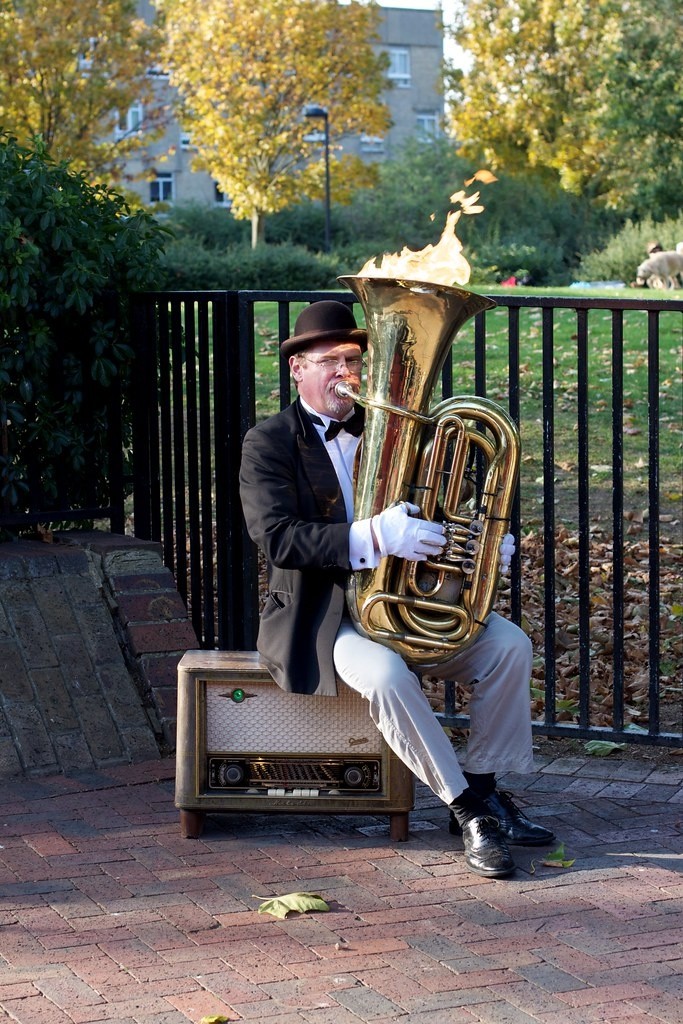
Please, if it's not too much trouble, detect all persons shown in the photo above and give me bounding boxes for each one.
[630,242,683,289]
[240,300,556,876]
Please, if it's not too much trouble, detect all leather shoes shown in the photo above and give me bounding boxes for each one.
[462,811,516,877]
[483,790,557,845]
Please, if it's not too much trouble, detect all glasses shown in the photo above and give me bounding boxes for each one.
[299,355,368,373]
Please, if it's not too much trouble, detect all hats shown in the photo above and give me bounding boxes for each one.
[280,299,369,362]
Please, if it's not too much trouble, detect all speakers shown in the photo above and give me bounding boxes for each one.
[174,648,414,812]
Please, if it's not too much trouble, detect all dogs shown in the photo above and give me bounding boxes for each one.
[636,242,683,290]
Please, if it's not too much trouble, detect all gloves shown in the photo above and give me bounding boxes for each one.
[499,534,516,574]
[372,501,448,561]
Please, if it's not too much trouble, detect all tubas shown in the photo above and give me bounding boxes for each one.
[329,274,523,668]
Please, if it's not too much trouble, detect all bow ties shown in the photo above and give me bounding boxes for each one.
[304,409,364,442]
